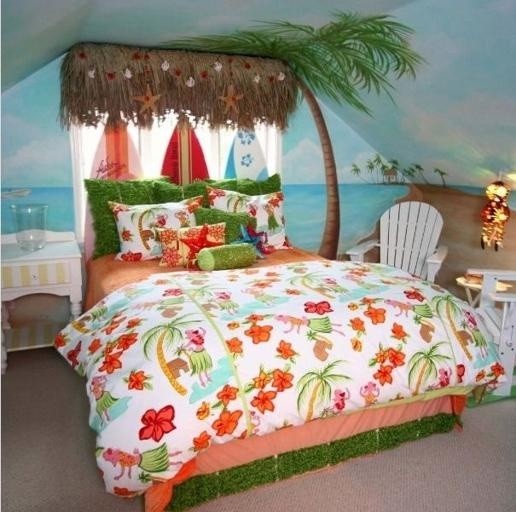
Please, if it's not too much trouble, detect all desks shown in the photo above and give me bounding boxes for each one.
[456,275,508,309]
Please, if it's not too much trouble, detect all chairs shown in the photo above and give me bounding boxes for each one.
[344,200,450,284]
[472,271,516,397]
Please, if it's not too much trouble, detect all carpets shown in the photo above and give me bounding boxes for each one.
[465,367,516,409]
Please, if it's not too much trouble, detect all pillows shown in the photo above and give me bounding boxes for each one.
[82,173,288,273]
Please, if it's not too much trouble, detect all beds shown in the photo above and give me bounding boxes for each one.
[86,247,478,510]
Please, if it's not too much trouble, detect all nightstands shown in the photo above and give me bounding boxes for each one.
[0,228,82,376]
